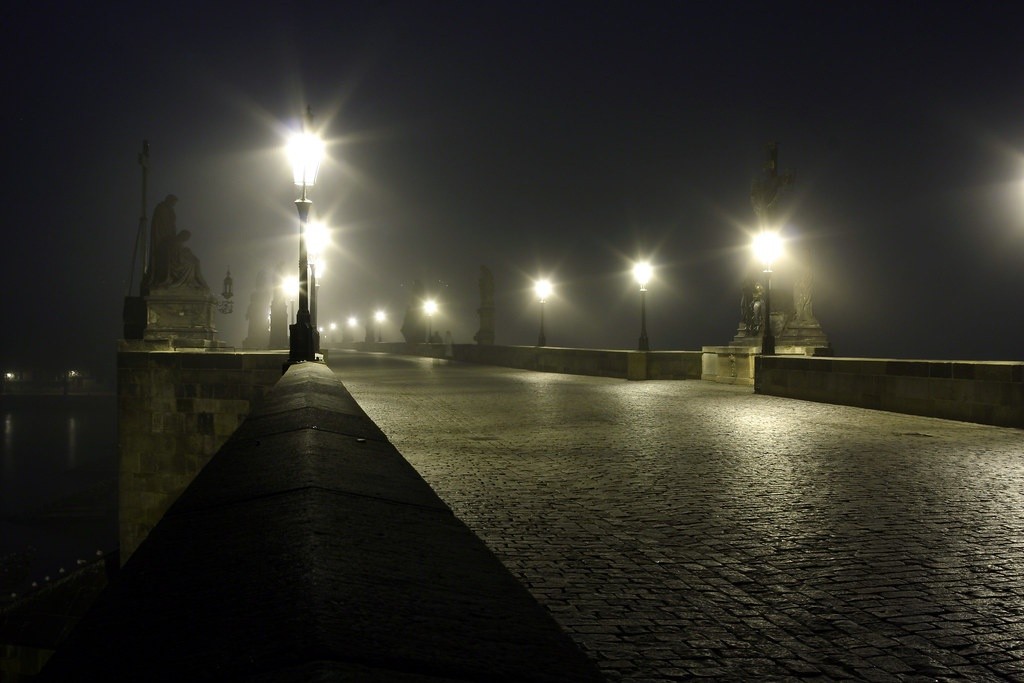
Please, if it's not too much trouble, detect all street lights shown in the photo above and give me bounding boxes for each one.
[287,222,325,354]
[533,278,553,347]
[282,130,326,361]
[422,300,437,344]
[751,229,785,356]
[374,310,385,343]
[631,260,654,351]
